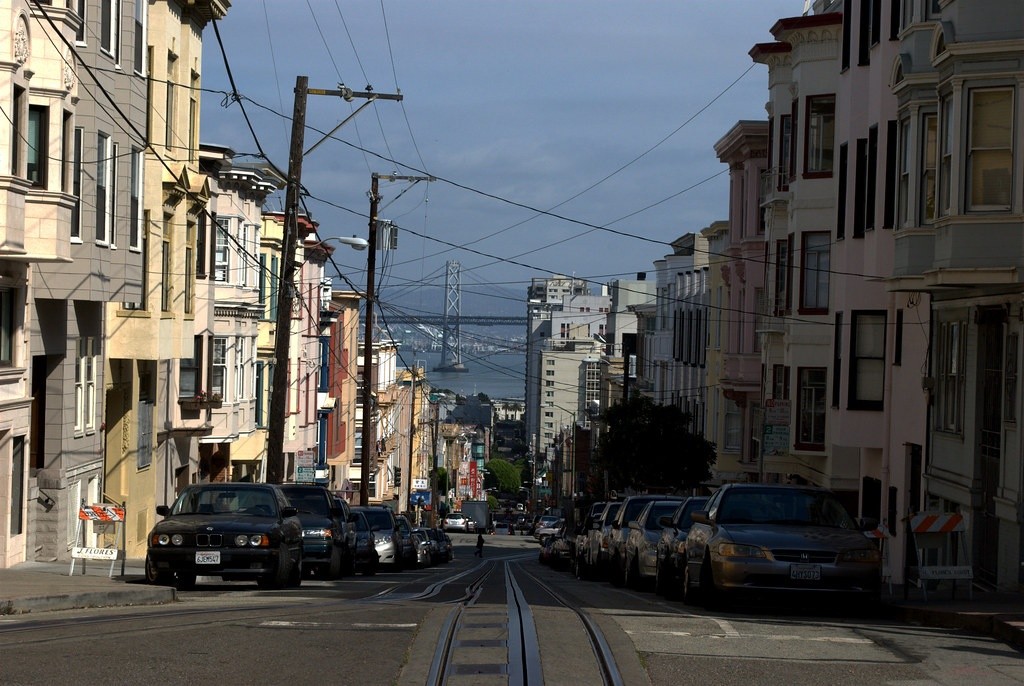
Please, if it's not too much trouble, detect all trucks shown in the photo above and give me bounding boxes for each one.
[460,500,494,534]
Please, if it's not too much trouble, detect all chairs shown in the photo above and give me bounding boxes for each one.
[197,503,214,512]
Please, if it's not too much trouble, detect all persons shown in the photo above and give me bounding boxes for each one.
[474,534,485,558]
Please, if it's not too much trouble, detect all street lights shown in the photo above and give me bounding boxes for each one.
[264,237,368,490]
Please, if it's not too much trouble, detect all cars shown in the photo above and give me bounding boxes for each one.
[516,502,523,511]
[145,482,304,592]
[536,477,881,623]
[517,516,524,522]
[271,480,455,582]
[442,512,480,533]
[525,520,532,529]
